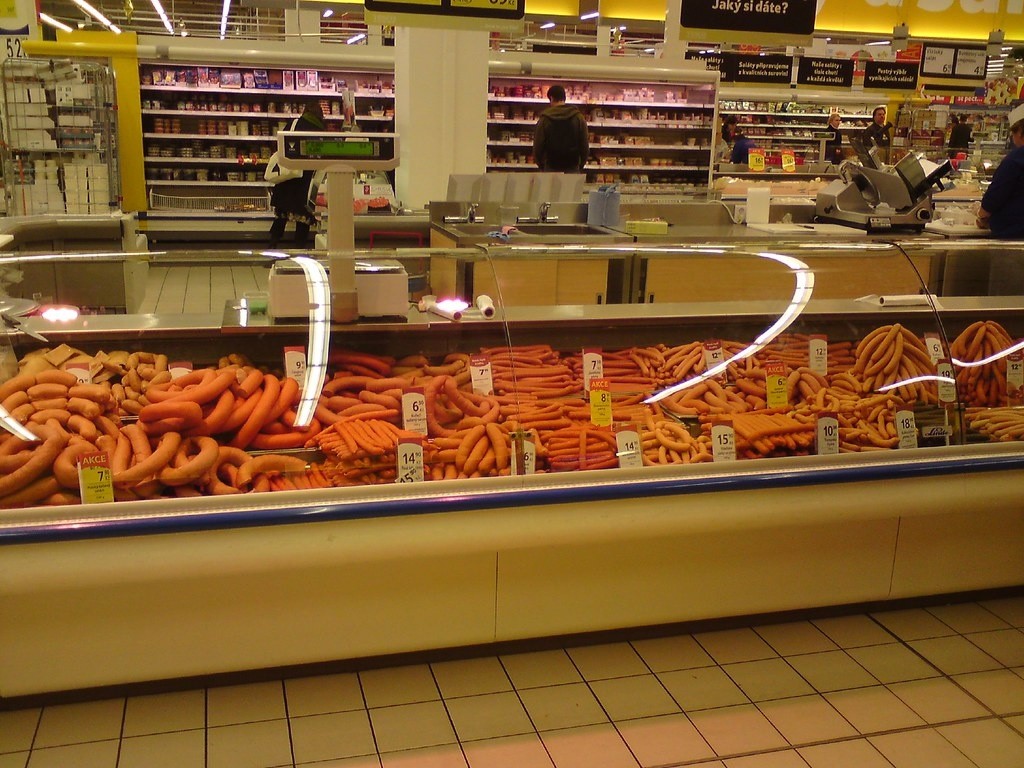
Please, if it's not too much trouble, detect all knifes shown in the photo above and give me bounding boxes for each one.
[797,225,814,229]
[1,313,49,342]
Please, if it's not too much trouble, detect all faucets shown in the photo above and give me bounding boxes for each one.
[517,201,558,223]
[443,201,485,224]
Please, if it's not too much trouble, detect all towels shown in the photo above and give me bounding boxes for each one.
[483,225,518,241]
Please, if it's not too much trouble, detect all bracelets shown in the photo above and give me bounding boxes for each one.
[977,208,988,224]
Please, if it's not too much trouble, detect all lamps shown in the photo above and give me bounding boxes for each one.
[987,30,1005,57]
[579,0,600,21]
[178,17,184,25]
[793,47,804,58]
[892,24,909,52]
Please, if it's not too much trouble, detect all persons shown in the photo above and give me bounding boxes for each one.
[944,114,975,159]
[532,84,589,173]
[721,115,757,164]
[382,114,395,195]
[862,107,890,169]
[817,114,842,165]
[976,101,1024,240]
[264,102,327,249]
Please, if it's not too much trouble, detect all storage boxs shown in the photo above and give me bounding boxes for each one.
[895,107,947,147]
[0,60,115,216]
[626,220,669,235]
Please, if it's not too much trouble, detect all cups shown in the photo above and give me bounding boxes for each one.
[746,187,770,224]
[244,292,269,313]
[500,205,519,227]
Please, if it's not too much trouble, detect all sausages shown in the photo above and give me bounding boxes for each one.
[0,321,1024,514]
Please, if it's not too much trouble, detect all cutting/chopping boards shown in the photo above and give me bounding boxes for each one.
[925,224,991,233]
[747,224,867,234]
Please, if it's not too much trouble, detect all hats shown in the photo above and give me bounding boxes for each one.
[1008,103,1024,128]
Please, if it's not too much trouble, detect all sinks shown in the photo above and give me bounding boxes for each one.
[452,225,520,235]
[512,225,612,235]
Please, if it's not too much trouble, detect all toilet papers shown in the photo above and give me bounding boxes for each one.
[746,187,770,223]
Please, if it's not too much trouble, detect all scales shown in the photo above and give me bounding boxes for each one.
[267,129,410,323]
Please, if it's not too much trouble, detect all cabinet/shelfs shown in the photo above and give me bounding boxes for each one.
[141,85,395,213]
[720,110,886,154]
[947,120,1010,147]
[487,98,715,195]
[463,245,1024,308]
[0,55,114,217]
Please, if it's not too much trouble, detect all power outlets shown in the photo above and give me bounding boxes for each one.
[734,205,746,225]
